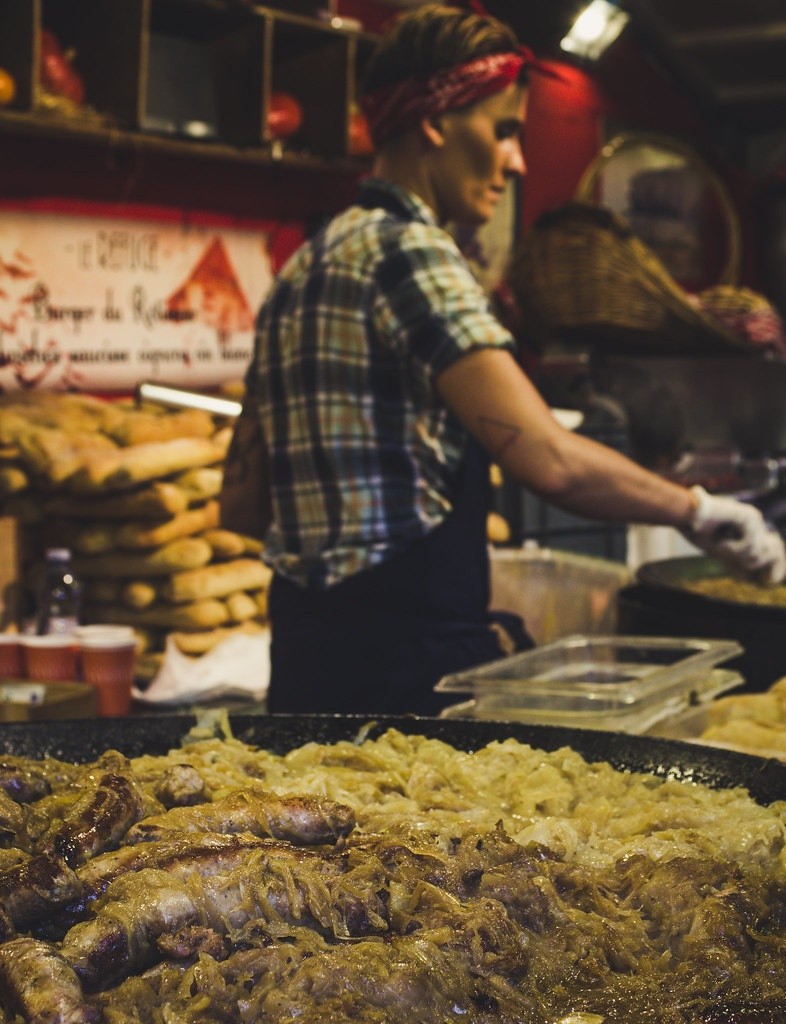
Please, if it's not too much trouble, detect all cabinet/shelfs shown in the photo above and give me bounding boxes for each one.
[0,1,376,180]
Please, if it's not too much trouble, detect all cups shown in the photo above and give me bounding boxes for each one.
[1,635,28,681]
[82,634,137,716]
[26,634,81,717]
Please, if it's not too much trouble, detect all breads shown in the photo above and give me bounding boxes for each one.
[0,377,511,679]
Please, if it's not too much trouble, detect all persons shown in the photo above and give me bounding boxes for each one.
[220,0,786,716]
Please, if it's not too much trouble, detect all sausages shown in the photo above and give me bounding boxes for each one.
[0,751,452,1024]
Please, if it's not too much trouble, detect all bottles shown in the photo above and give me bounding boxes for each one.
[35,549,81,634]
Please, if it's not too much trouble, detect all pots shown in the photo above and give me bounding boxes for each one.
[637,555,786,628]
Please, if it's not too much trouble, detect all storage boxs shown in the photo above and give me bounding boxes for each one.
[430,632,745,733]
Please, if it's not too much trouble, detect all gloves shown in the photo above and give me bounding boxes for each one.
[680,483,786,586]
